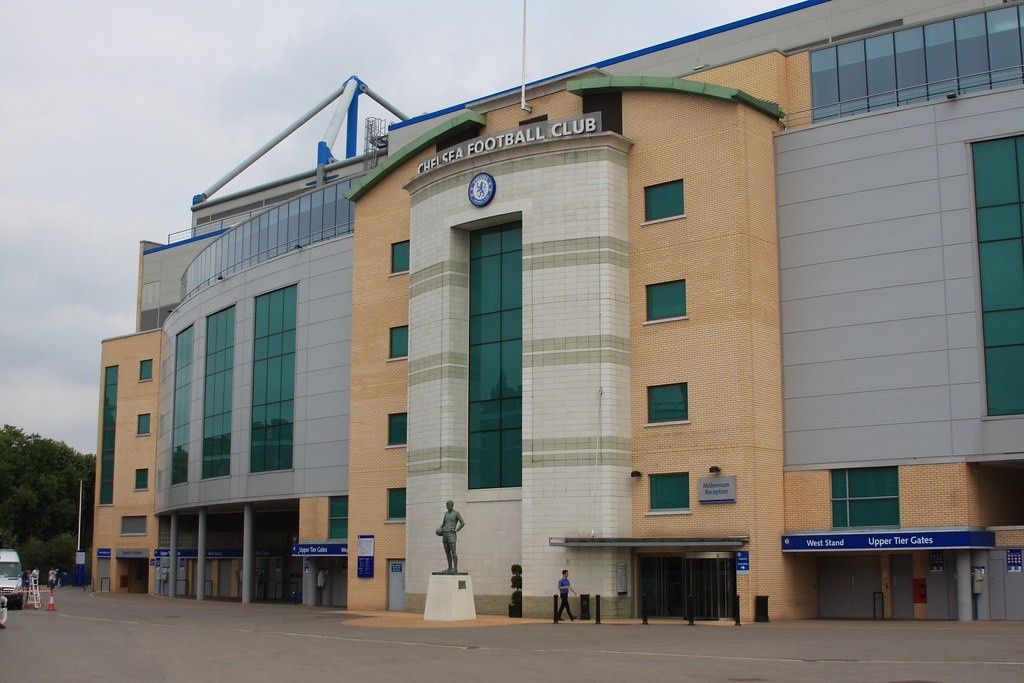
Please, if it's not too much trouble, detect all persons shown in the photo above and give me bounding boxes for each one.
[436,499,465,572]
[26,568,39,588]
[557,570,577,621]
[48,567,57,589]
[60,570,67,587]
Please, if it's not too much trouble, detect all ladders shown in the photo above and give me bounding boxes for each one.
[24,570,42,611]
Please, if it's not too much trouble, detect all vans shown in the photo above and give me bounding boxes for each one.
[0,548,27,610]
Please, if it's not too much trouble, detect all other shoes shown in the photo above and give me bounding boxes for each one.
[558,618,563,621]
[571,616,577,621]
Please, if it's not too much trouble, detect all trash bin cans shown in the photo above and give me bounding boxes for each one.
[580,594,591,620]
[755,595,770,622]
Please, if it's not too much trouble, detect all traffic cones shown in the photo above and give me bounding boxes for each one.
[46,589,58,612]
